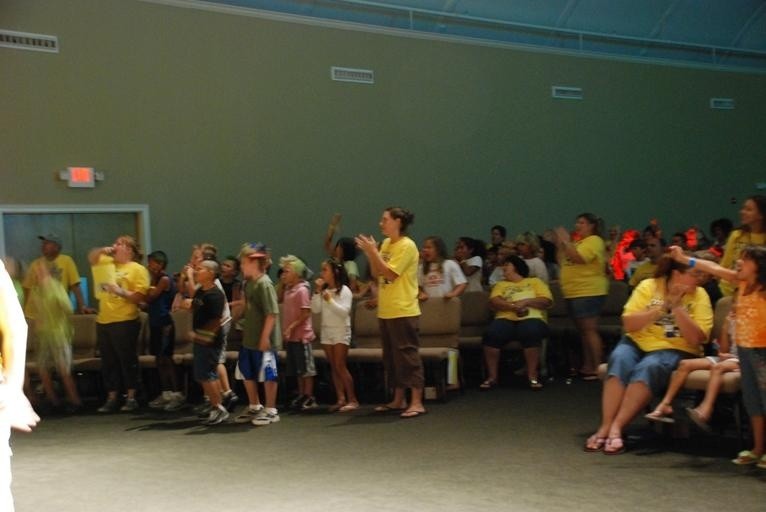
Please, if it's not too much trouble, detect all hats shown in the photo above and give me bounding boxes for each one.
[38,233,63,245]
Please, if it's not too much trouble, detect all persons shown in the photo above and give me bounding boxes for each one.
[235,240,285,426]
[354,206,428,418]
[274,255,319,411]
[87,236,151,413]
[479,255,555,390]
[583,249,714,456]
[556,212,609,380]
[514,229,549,378]
[0,231,97,512]
[138,244,236,427]
[415,224,643,302]
[310,256,360,412]
[644,192,766,471]
[236,212,380,314]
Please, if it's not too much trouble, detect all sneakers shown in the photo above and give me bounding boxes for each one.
[97,390,359,426]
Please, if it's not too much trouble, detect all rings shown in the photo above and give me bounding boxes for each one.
[512,303,514,306]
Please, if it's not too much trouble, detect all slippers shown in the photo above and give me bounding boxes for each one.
[374,405,406,412]
[479,378,496,390]
[528,376,542,389]
[399,408,427,418]
[583,401,765,469]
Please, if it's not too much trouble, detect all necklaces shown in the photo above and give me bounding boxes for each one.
[115,263,124,273]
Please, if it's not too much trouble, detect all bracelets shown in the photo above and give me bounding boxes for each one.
[120,288,125,298]
